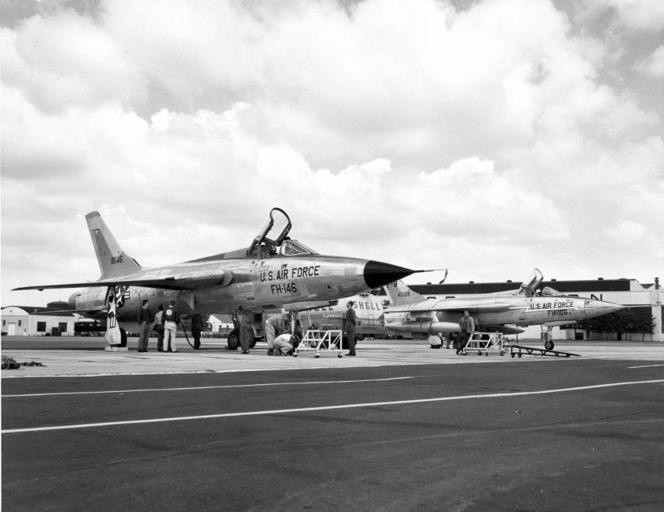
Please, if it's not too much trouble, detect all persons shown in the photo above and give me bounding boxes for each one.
[289,319,302,341]
[237,304,254,354]
[343,300,356,357]
[455,310,475,355]
[137,300,151,352]
[310,323,324,348]
[446,332,457,349]
[150,303,165,352]
[190,312,203,350]
[159,299,179,352]
[272,333,300,355]
[264,312,291,356]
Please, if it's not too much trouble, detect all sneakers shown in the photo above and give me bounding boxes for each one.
[267,348,294,356]
[346,353,353,356]
[158,347,178,353]
[138,349,147,352]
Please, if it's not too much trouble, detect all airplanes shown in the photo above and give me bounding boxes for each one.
[10,207,426,351]
[282,268,634,356]
